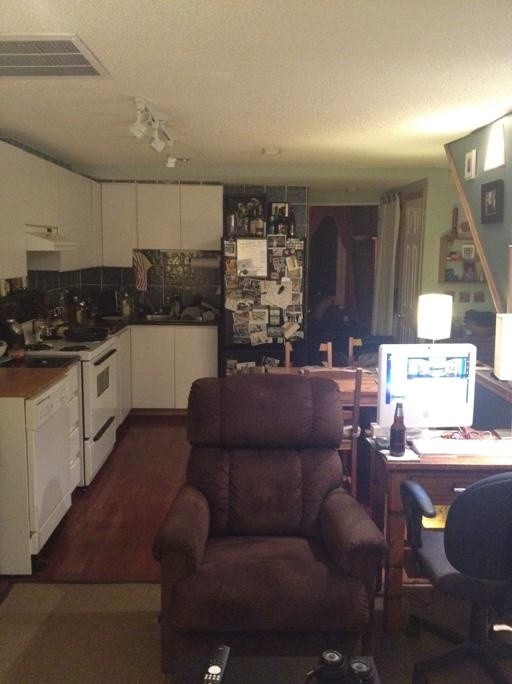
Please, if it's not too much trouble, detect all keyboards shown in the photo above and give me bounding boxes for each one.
[410,438,512,457]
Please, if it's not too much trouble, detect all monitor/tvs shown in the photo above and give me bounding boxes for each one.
[378,343,477,440]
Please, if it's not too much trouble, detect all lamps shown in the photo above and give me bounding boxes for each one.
[127,98,177,171]
[494,313,512,382]
[415,292,453,342]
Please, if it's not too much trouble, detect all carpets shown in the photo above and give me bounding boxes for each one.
[1,578,510,684]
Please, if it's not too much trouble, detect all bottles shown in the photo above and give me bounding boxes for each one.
[389,403,407,457]
[173,295,180,320]
[73,295,89,325]
[120,293,132,320]
[249,204,296,236]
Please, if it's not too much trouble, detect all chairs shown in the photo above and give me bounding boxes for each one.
[399,472,512,668]
[284,342,332,366]
[303,368,363,501]
[347,336,395,368]
[152,374,390,680]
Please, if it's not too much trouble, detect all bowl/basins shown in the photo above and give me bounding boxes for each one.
[64,326,108,341]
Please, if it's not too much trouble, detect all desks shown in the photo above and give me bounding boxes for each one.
[268,365,380,407]
[366,424,512,629]
[171,651,382,684]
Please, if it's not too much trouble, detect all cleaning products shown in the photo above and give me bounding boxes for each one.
[123,293,132,316]
[173,294,182,318]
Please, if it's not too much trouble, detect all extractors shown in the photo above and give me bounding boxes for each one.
[25,224,77,253]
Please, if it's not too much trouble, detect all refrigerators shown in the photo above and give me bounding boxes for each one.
[216,235,308,379]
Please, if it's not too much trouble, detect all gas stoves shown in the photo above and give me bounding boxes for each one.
[17,331,117,360]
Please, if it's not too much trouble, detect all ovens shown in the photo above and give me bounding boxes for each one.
[81,345,118,490]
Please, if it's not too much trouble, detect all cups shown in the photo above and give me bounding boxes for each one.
[1,341,6,358]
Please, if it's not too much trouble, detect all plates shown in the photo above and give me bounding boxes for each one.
[0,354,12,364]
